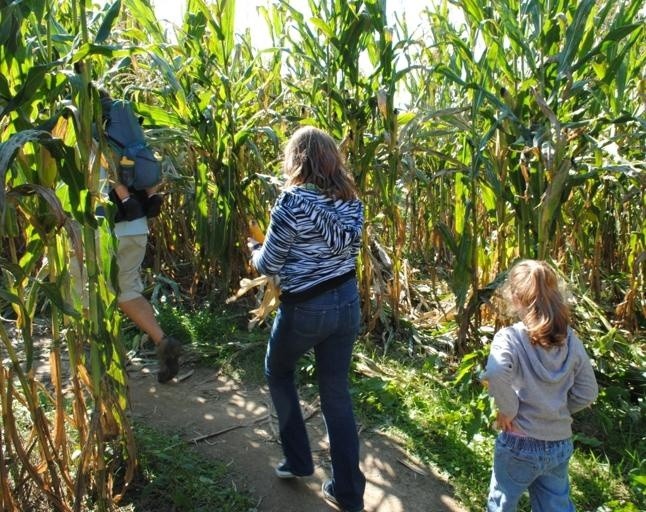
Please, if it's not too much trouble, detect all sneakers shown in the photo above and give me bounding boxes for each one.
[321,479,364,511]
[122,198,140,221]
[275,462,313,478]
[158,337,181,382]
[147,194,161,218]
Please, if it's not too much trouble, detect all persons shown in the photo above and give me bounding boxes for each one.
[57,89,181,384]
[248,126,365,512]
[486,260,598,512]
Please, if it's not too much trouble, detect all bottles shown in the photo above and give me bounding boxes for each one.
[118,153,137,187]
